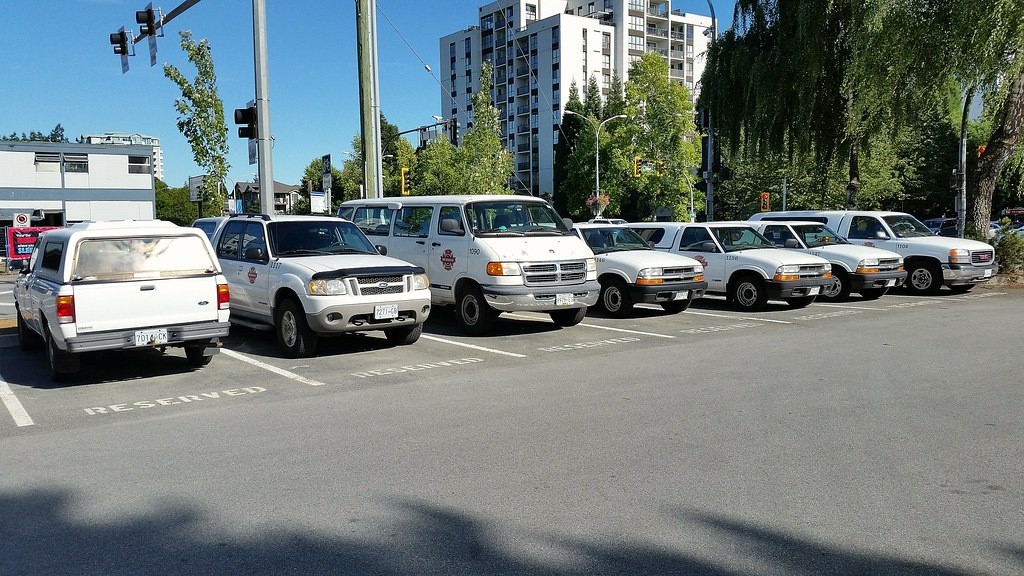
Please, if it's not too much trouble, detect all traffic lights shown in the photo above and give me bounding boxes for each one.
[401,167,411,195]
[760,192,769,211]
[451,118,460,142]
[110,31,128,55]
[419,126,427,150]
[978,145,986,157]
[136,9,155,36]
[702,135,720,173]
[235,107,256,139]
[656,160,664,177]
[634,156,642,177]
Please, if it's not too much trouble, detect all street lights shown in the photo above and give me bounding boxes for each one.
[564,109,627,219]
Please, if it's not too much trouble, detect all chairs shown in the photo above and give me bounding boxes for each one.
[493,214,510,229]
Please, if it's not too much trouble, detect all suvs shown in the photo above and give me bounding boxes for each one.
[923,217,958,238]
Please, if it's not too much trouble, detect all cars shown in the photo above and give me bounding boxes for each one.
[588,219,628,226]
[987,219,1024,243]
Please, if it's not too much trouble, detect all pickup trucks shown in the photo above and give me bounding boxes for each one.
[12,218,230,382]
[510,217,708,318]
[748,211,999,295]
[191,213,431,358]
[628,222,834,311]
[715,222,908,303]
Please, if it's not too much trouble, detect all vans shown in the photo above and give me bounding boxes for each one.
[337,194,602,335]
